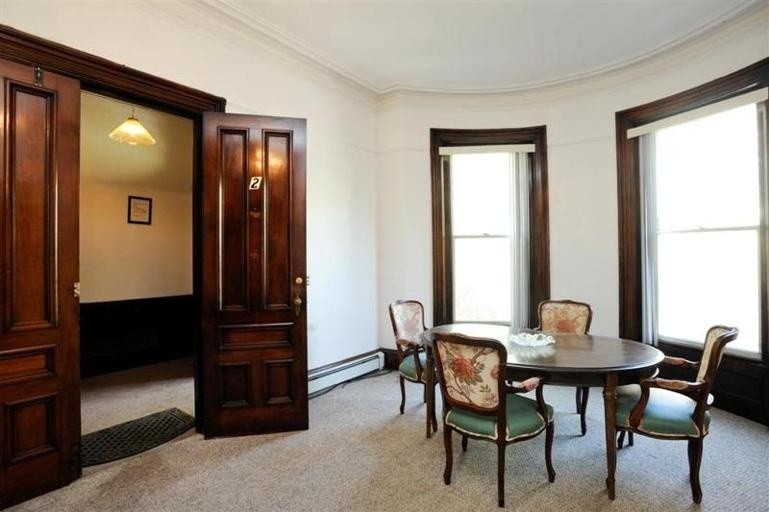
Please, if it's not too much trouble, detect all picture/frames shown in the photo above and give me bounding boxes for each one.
[125,195,152,226]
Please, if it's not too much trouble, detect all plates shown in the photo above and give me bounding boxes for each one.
[506,345,556,359]
[504,328,553,345]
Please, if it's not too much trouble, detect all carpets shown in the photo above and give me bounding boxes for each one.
[77,407,195,463]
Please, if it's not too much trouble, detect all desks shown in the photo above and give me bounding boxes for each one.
[425,322,667,486]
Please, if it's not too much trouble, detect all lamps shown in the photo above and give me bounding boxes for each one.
[106,106,157,148]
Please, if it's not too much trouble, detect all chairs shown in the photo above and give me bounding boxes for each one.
[604,321,742,505]
[529,298,595,436]
[427,330,559,508]
[388,296,443,432]
